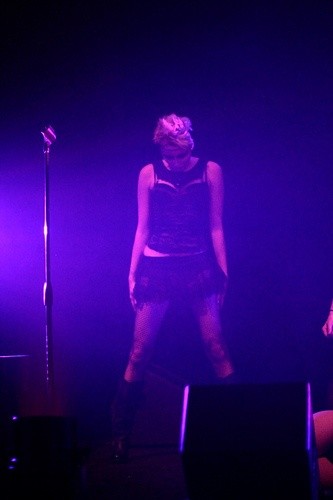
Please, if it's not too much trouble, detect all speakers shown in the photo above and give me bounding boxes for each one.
[177,380,321,500]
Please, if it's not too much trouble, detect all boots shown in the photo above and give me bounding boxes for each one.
[111,381,143,464]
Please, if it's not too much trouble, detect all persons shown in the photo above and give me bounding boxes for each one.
[108,113,233,463]
